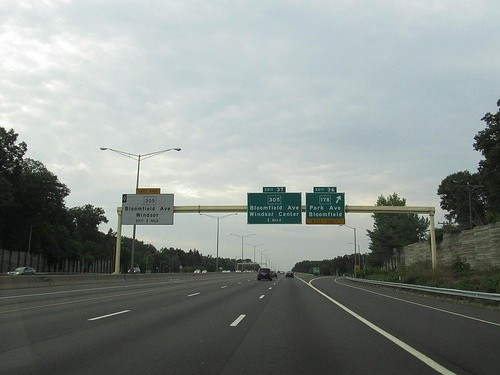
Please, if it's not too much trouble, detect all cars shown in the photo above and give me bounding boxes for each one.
[7,267,36,276]
[286,271,294,278]
[128,268,141,273]
[194,270,207,274]
[271,271,277,278]
[280,271,284,274]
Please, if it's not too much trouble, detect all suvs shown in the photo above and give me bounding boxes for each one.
[257,267,273,281]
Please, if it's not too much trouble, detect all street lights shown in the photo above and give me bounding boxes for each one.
[246,243,264,271]
[230,233,256,272]
[146,254,154,272]
[200,213,238,271]
[160,261,167,272]
[26,222,47,266]
[99,147,181,273]
[340,225,357,277]
[113,242,124,272]
[349,243,361,271]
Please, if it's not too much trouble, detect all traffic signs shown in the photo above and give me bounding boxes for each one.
[305,192,346,225]
